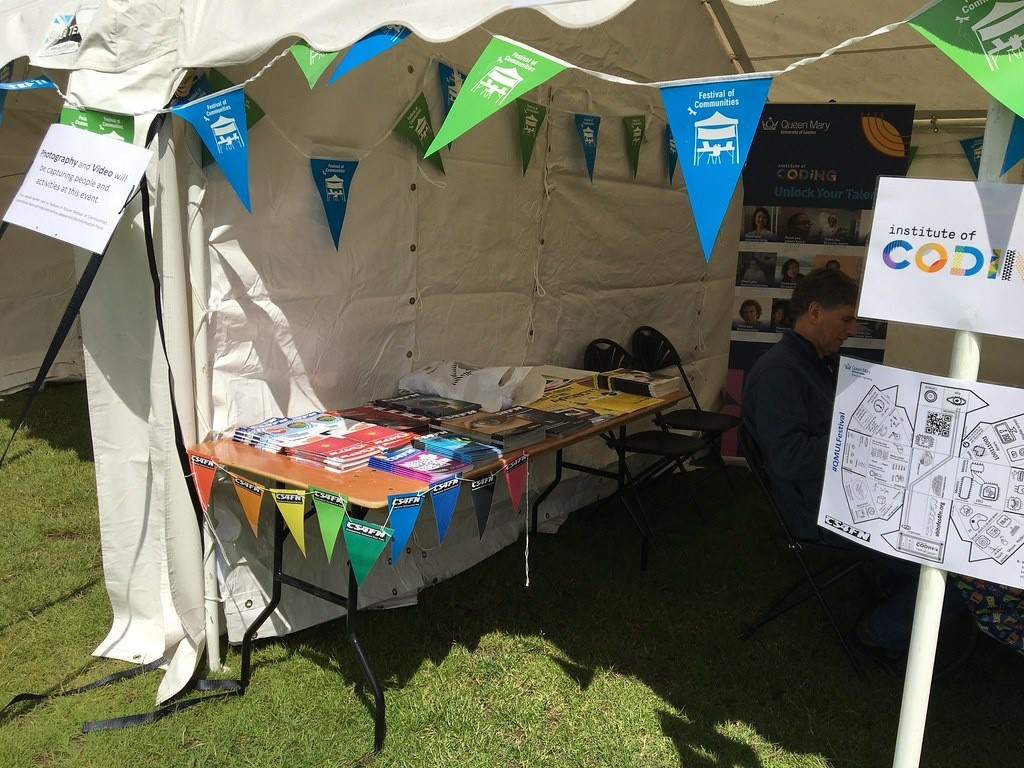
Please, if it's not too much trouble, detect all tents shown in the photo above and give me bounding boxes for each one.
[180,0,1024,768]
[0,0,205,705]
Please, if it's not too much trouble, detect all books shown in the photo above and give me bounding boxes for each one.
[231,364,680,484]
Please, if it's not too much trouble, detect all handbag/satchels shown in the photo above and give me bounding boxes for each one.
[399,360,548,411]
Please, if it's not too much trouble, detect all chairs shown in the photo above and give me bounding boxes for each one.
[734,426,869,681]
[585,337,707,549]
[631,325,741,516]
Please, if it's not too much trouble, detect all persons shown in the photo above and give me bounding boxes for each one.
[771,307,784,329]
[741,207,777,242]
[826,260,841,271]
[778,258,806,289]
[819,214,848,244]
[742,257,766,283]
[738,268,976,686]
[739,299,763,331]
[782,213,814,244]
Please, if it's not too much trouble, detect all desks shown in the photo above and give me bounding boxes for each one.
[932,572,1024,683]
[189,381,691,761]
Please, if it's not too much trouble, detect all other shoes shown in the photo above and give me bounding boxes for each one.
[842,624,904,680]
